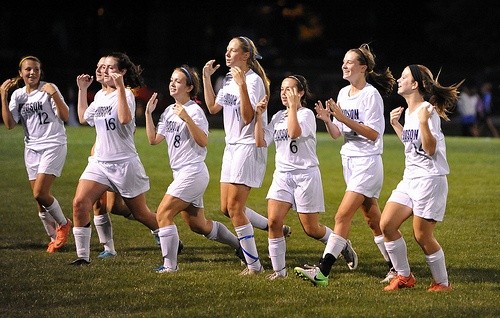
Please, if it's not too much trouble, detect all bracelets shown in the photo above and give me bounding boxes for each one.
[50,91,57,96]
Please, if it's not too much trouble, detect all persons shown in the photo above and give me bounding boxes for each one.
[379,64,449,292]
[202,36,291,275]
[144,66,248,272]
[0,56,74,253]
[87,53,161,258]
[292,42,397,287]
[66,52,184,267]
[254,74,358,279]
[456,84,495,136]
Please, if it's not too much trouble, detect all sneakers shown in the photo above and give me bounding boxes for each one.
[340,238,358,271]
[97,251,118,259]
[427,281,451,293]
[154,265,179,273]
[237,266,265,276]
[265,272,289,281]
[46,241,57,253]
[53,218,74,249]
[379,268,398,284]
[384,273,416,290]
[293,265,329,287]
[68,258,91,266]
[280,225,291,239]
